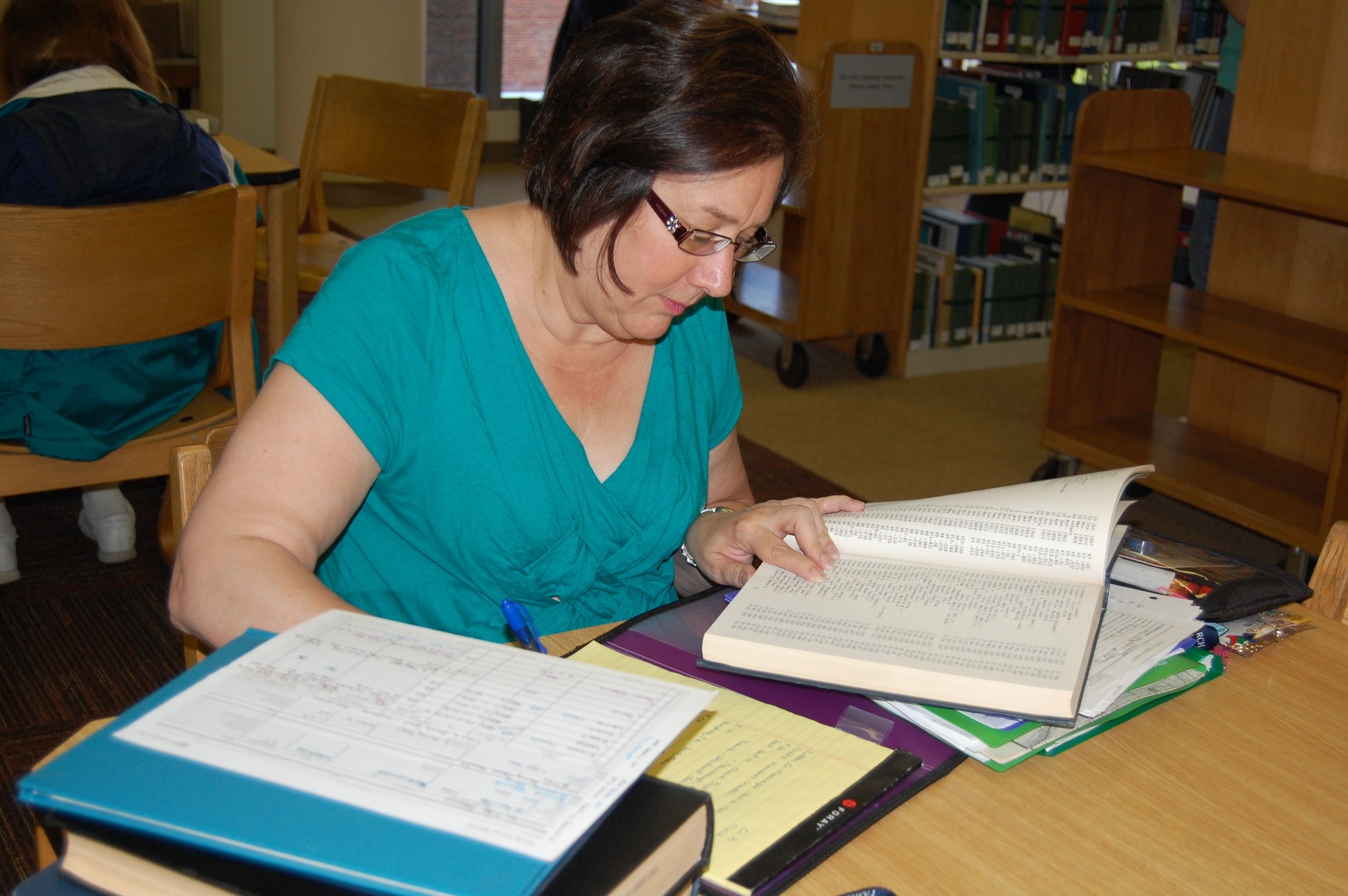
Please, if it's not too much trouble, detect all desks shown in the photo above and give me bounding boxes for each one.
[214,132,301,363]
[28,600,1348,896]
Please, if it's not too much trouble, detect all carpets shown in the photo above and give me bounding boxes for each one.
[0,428,862,896]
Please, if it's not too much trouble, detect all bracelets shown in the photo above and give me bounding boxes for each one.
[681,507,738,567]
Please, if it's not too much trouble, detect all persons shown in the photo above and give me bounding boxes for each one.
[170,5,866,652]
[0,0,264,582]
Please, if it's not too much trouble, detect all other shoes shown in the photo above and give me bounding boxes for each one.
[79,501,137,563]
[0,523,21,584]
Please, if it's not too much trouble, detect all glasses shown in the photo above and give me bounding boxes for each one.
[645,187,776,262]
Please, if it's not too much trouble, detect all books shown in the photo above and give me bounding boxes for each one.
[942,0,1225,56]
[927,63,1232,186]
[909,205,1064,348]
[18,609,919,896]
[694,463,1223,771]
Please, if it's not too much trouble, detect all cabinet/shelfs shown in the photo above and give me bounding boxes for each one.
[902,0,1229,377]
[1029,91,1348,579]
[706,0,930,388]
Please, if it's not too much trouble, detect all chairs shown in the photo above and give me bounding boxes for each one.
[0,183,255,557]
[251,75,490,292]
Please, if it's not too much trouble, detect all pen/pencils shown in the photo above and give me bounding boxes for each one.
[501,599,545,654]
